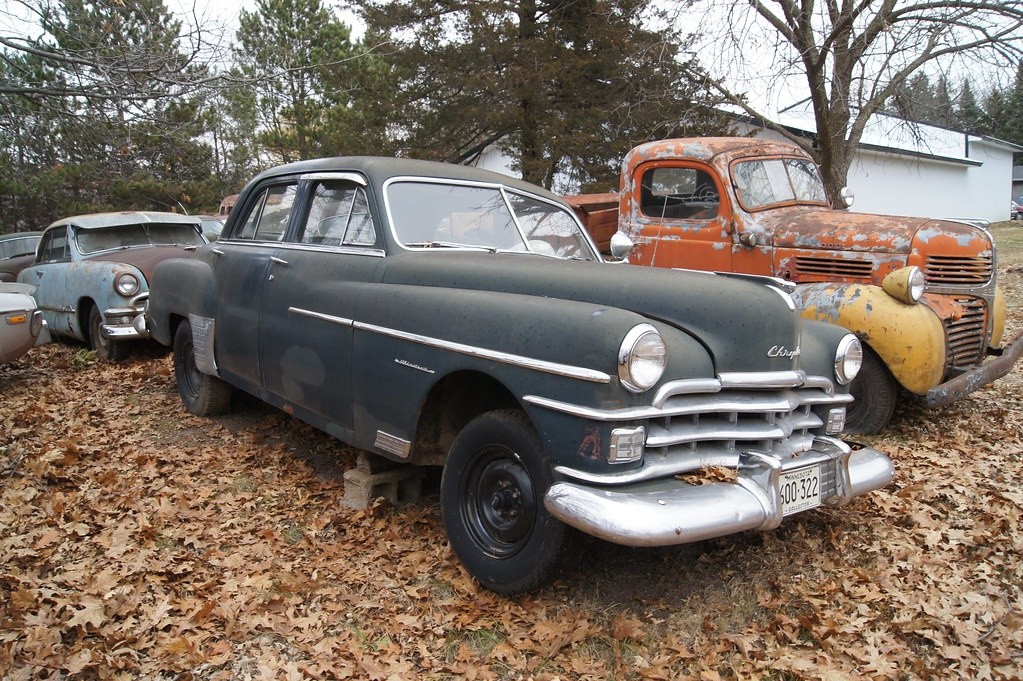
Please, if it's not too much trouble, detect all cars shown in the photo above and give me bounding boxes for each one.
[144,148,897,593]
[14,205,212,367]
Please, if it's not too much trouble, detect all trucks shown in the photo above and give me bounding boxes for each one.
[556,135,1010,440]
